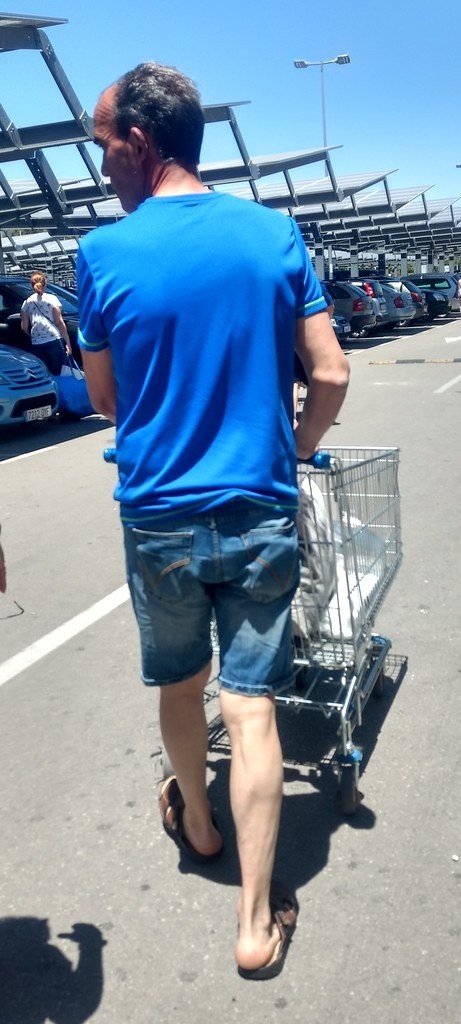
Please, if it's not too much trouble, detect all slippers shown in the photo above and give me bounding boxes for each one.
[159,775,224,863]
[238,888,296,980]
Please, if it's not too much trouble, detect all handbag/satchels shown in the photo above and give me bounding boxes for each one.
[53,353,95,416]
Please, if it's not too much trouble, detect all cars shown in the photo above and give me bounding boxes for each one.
[1,347,60,432]
[380,284,417,333]
[422,289,449,323]
[346,279,388,321]
[329,315,351,343]
[0,277,81,358]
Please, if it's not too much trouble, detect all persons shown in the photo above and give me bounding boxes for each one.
[74,61,350,980]
[458,276,461,316]
[21,274,71,423]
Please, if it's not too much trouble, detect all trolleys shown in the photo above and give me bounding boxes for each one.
[102,445,403,817]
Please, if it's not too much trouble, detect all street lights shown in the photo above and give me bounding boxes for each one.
[294,54,353,178]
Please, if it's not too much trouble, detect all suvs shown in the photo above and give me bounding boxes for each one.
[319,280,379,337]
[407,273,461,319]
[370,277,429,327]
